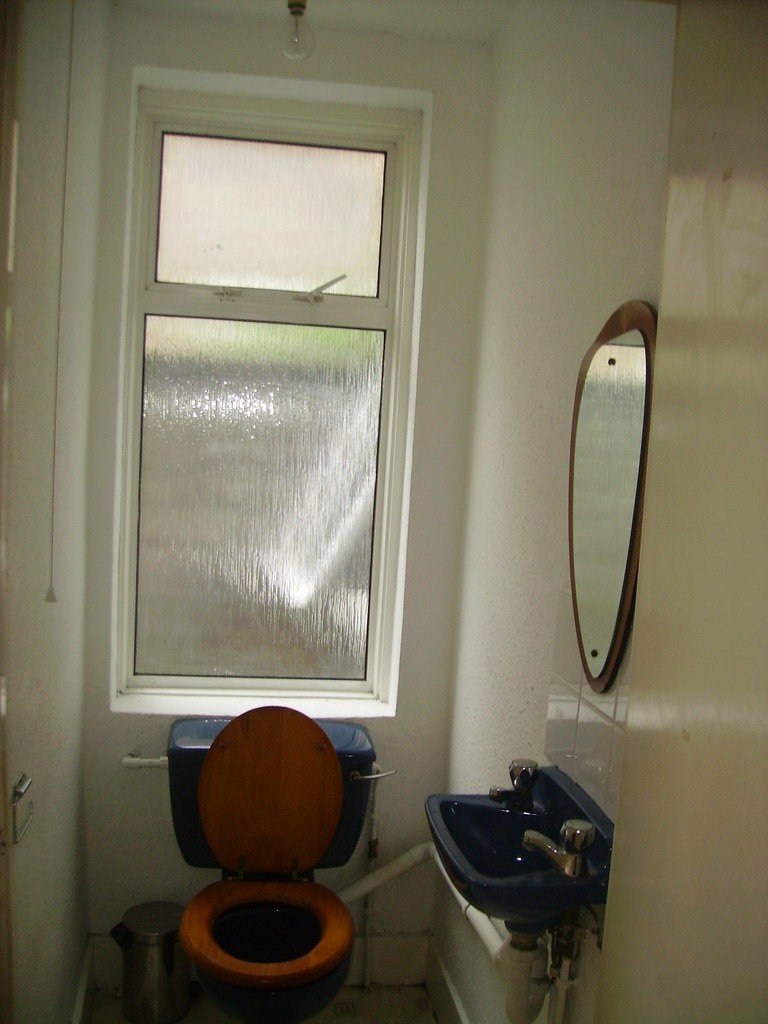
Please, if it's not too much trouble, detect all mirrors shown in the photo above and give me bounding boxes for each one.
[566,297,658,694]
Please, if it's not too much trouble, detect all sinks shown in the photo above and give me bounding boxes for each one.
[424,764,615,923]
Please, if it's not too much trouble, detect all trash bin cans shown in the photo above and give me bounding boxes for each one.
[108,900,192,1024]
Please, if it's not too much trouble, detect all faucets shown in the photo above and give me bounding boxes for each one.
[488,758,540,810]
[522,819,596,879]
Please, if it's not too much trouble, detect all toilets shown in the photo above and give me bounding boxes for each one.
[166,705,377,1024]
